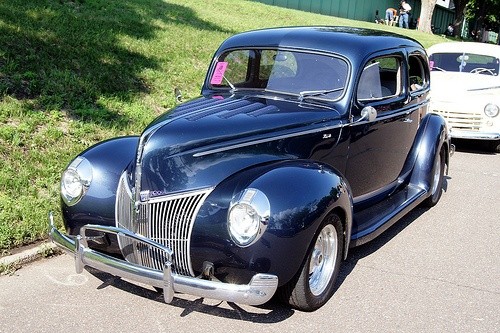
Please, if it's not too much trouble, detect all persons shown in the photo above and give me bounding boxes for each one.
[371,0,456,38]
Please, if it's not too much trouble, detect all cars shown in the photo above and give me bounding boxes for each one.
[47,25,455,312]
[423,41,500,154]
